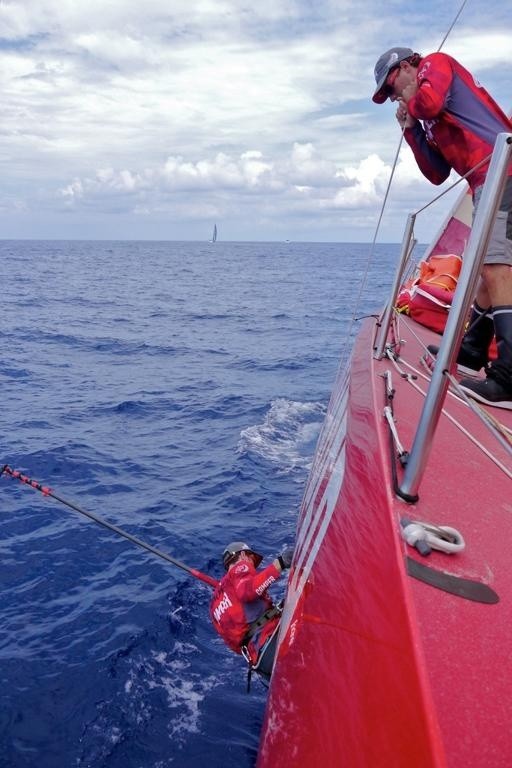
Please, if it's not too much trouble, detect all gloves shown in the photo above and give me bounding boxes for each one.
[278,549,294,571]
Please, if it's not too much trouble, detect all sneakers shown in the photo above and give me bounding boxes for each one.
[427,343,488,376]
[459,367,512,409]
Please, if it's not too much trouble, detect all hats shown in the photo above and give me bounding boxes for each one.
[223,542,263,569]
[371,47,414,104]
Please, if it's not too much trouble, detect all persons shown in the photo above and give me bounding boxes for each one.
[208,541,295,681]
[372,46,512,410]
